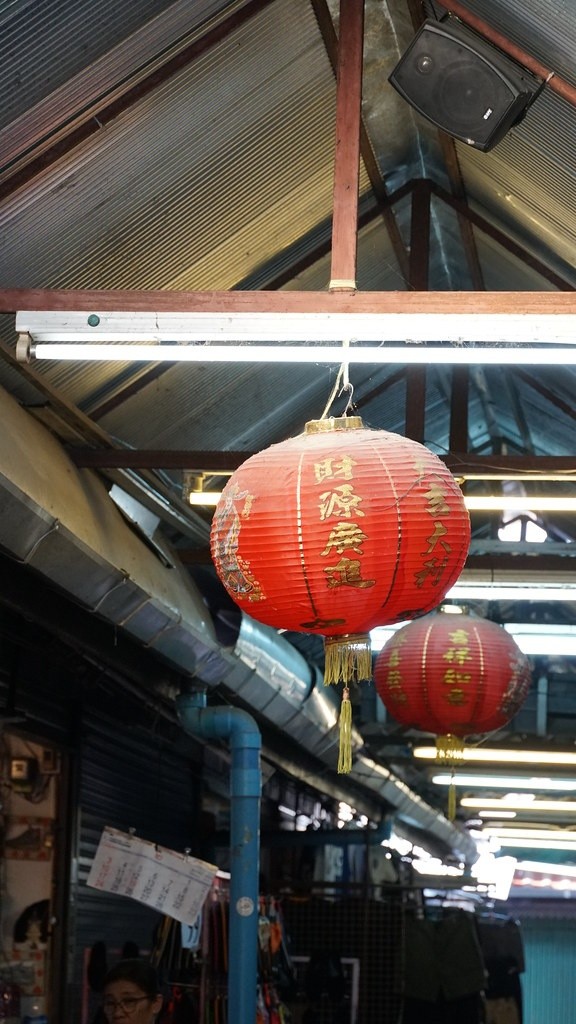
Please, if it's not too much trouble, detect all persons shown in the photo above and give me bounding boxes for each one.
[102,962,168,1024]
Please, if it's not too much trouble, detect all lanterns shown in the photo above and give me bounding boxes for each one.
[209,416,470,774]
[374,604,531,821]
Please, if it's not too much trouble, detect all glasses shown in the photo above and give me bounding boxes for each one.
[101,995,150,1015]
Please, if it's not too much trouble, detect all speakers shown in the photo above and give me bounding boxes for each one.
[390,10,553,153]
